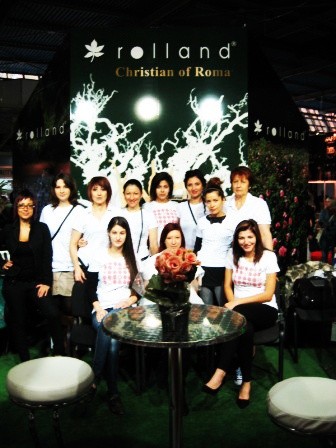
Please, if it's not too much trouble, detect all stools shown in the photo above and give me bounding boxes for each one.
[7,355,96,448]
[266,376,336,448]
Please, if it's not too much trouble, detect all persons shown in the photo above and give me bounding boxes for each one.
[0,191,55,362]
[78,179,159,269]
[206,306,223,325]
[127,306,147,323]
[221,163,275,267]
[139,170,186,255]
[63,215,149,423]
[106,313,122,327]
[189,305,204,323]
[140,222,205,417]
[191,176,237,311]
[174,167,205,256]
[221,310,244,333]
[69,177,131,359]
[316,196,336,266]
[37,175,86,358]
[202,218,283,412]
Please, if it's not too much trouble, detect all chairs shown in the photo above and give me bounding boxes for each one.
[253,279,298,382]
[55,282,98,356]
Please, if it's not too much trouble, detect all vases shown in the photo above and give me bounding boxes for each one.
[159,302,191,341]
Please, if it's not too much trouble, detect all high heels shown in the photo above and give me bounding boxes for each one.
[107,393,124,415]
[79,385,97,404]
[237,390,250,409]
[202,381,222,395]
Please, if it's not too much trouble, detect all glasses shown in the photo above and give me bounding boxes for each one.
[17,205,35,209]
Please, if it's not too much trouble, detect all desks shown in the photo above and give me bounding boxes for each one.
[101,305,246,448]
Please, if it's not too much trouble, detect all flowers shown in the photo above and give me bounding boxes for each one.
[244,142,316,257]
[145,247,201,305]
